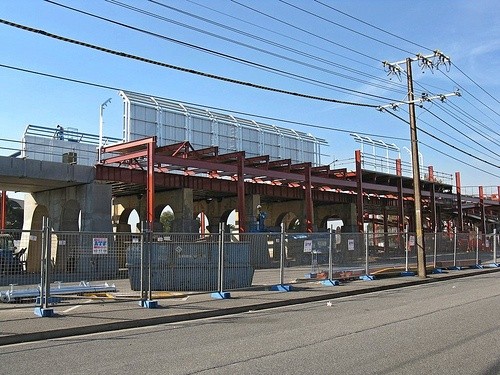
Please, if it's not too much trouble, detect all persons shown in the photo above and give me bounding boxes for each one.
[57,125,64,140]
[8,247,17,264]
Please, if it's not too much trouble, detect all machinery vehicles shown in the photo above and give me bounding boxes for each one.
[0,234,28,274]
